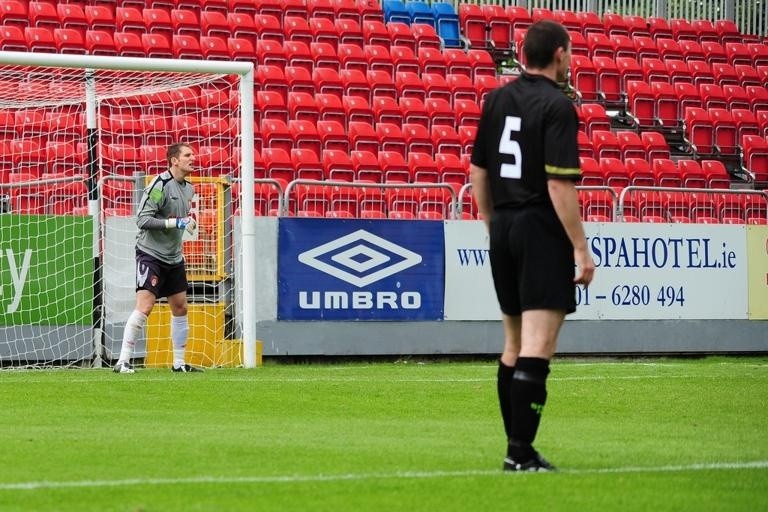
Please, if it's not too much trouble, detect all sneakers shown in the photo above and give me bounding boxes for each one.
[171,363,205,373]
[502,451,556,472]
[113,361,135,375]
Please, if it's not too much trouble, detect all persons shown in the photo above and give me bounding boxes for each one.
[467,21,595,474]
[113,143,202,374]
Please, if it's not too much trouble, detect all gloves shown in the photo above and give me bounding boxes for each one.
[167,216,197,235]
